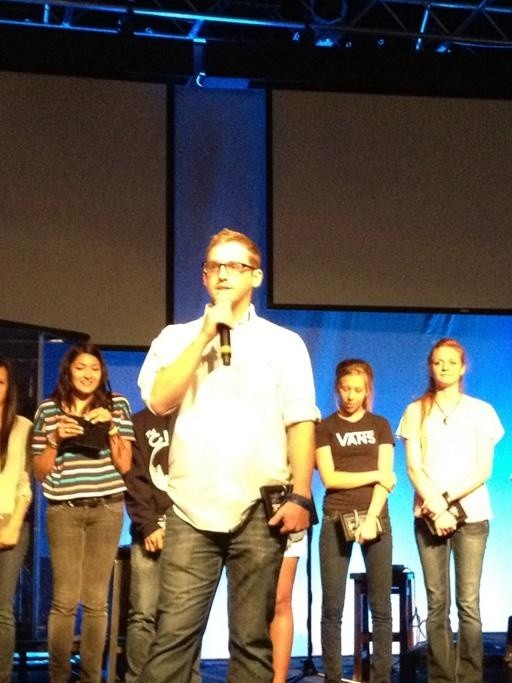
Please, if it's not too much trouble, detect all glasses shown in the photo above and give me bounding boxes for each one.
[201,260,255,277]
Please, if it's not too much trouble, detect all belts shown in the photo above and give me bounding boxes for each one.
[44,494,125,509]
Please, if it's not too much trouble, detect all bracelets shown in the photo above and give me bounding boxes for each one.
[287,493,313,511]
[108,424,118,436]
[442,492,452,506]
[46,432,60,449]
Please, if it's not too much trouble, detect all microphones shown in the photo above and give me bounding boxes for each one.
[215,292,233,365]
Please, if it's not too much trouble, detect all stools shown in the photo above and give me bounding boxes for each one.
[353,570,414,683]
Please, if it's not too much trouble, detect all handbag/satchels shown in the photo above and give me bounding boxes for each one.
[58,412,114,452]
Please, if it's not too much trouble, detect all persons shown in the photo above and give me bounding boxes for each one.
[126,406,202,683]
[32,344,136,683]
[269,529,306,683]
[316,358,397,683]
[137,228,322,683]
[0,356,35,683]
[396,338,505,683]
